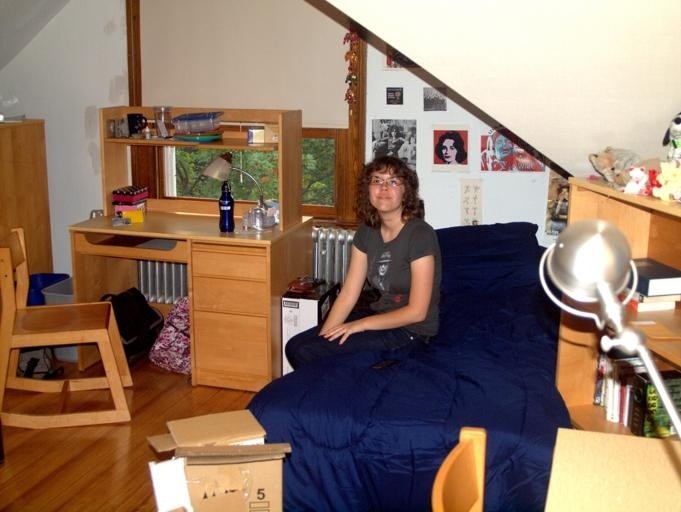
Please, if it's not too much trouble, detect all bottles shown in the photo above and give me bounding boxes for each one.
[155,106,173,138]
[248,207,253,229]
[253,208,263,231]
[145,126,151,140]
[218,180,236,234]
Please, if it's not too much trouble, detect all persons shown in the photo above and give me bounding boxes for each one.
[288,155,446,369]
[434,132,467,165]
[383,125,403,163]
[550,185,569,233]
[481,131,515,170]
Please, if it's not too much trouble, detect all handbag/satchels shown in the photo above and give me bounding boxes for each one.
[100,286,163,360]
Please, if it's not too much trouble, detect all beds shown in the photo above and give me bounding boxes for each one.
[245,221,572,512]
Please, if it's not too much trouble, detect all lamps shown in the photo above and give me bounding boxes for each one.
[538,218,680,442]
[202,150,276,230]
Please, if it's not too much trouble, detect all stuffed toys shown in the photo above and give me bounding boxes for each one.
[579,112,681,207]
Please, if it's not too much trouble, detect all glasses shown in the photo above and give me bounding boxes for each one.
[370,176,402,188]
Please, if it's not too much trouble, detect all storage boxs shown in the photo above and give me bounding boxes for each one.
[41,277,74,306]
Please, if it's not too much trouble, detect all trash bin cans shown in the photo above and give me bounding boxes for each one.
[46,276,78,362]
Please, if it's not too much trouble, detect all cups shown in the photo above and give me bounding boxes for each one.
[128,112,147,136]
[153,106,171,138]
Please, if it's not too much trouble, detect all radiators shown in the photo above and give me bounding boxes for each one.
[311,226,360,283]
[139,259,188,305]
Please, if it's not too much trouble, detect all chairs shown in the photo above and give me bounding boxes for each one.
[431,427,488,511]
[0,225,134,429]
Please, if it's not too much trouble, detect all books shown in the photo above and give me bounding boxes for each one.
[618,257,681,313]
[588,345,681,440]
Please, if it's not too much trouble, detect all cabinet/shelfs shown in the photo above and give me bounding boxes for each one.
[556,176,681,432]
[0,119,56,277]
[68,106,315,393]
